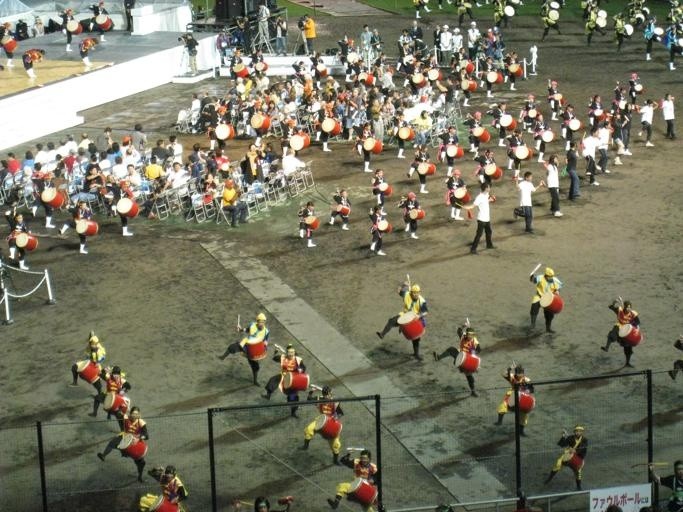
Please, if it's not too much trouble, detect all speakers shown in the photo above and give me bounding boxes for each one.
[227,0,244,16]
[216,0,227,22]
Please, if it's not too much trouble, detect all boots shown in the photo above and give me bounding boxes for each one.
[7,58,14,67]
[376,323,584,490]
[69,372,145,484]
[26,68,36,80]
[191,0,683,256]
[62,23,107,72]
[528,314,679,380]
[216,349,342,510]
[8,206,133,270]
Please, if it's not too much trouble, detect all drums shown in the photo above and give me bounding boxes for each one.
[596,10,608,28]
[321,117,341,137]
[593,108,606,120]
[529,109,543,122]
[653,28,665,42]
[619,99,628,110]
[508,391,536,412]
[290,132,310,151]
[378,182,393,197]
[378,220,393,232]
[215,124,234,140]
[542,131,557,143]
[499,6,514,19]
[634,84,644,94]
[66,20,82,34]
[500,114,517,131]
[540,292,563,314]
[473,127,491,143]
[363,137,383,155]
[618,325,643,345]
[484,163,502,179]
[623,7,650,37]
[77,359,182,512]
[304,216,320,229]
[15,188,139,251]
[454,187,471,204]
[567,119,583,131]
[250,114,271,130]
[397,312,425,341]
[412,72,427,88]
[461,80,478,91]
[563,451,585,470]
[456,351,480,372]
[1,36,18,53]
[255,62,268,72]
[412,59,523,91]
[555,94,565,107]
[416,163,436,175]
[410,209,425,220]
[233,64,248,78]
[516,146,534,160]
[96,14,111,30]
[397,126,415,141]
[336,204,351,218]
[548,2,560,20]
[246,336,380,505]
[358,72,374,86]
[446,144,464,158]
[316,64,327,77]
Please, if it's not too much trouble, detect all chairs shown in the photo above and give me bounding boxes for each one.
[0,135,314,226]
[166,77,468,155]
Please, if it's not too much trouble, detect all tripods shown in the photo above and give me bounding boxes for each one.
[179,46,189,73]
[292,31,309,56]
